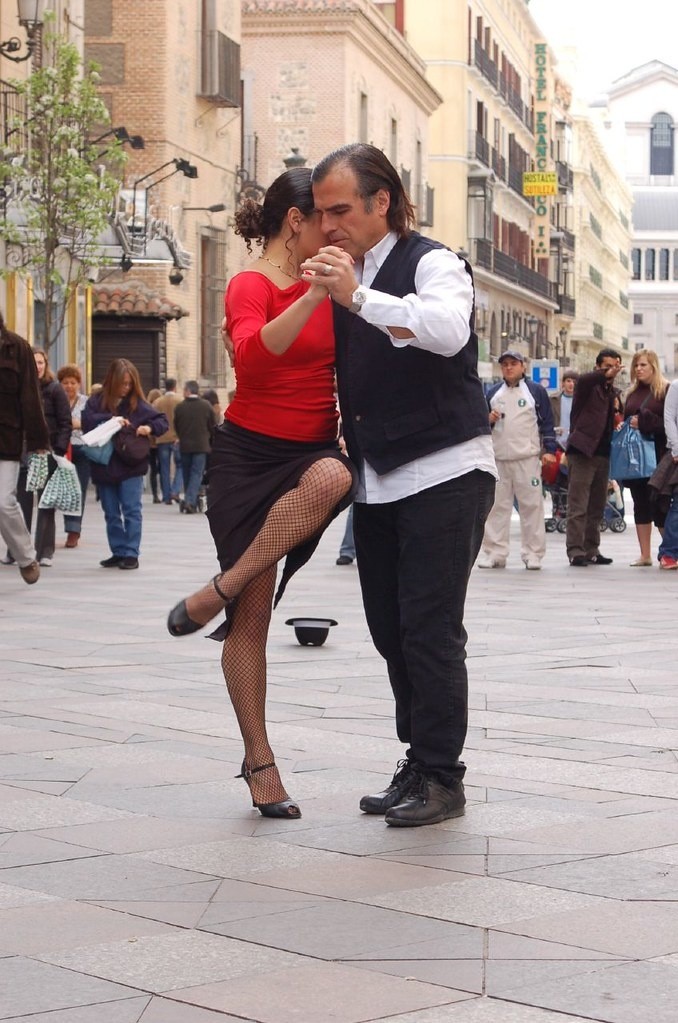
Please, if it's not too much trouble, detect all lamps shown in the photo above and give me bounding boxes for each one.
[143,165,199,238]
[21,236,60,266]
[87,135,145,164]
[183,203,227,212]
[510,315,538,342]
[168,268,184,285]
[542,327,567,351]
[0,0,45,62]
[78,126,130,152]
[96,253,134,282]
[131,158,190,250]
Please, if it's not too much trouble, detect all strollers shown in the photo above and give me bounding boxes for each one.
[541,437,628,533]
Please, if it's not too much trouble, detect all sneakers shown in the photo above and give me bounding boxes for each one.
[569,555,612,565]
[660,554,678,569]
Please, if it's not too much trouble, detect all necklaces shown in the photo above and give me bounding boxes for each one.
[258,256,299,281]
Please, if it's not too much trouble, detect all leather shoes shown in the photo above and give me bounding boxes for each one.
[359,760,467,827]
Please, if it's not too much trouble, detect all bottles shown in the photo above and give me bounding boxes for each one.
[494,401,506,431]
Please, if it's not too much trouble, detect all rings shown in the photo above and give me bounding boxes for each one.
[324,265,333,274]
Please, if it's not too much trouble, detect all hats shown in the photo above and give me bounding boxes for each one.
[498,351,525,364]
[563,370,578,379]
[285,618,337,646]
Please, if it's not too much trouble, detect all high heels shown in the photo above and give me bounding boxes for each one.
[169,491,183,504]
[233,754,302,819]
[167,572,237,637]
[180,499,203,514]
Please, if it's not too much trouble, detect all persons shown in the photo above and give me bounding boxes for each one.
[0,309,52,585]
[0,347,72,566]
[301,141,500,827]
[336,422,356,566]
[90,378,236,515]
[57,363,89,549]
[549,349,678,570]
[81,359,170,570]
[167,167,359,819]
[478,351,556,570]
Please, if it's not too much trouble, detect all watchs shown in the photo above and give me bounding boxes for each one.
[348,284,367,314]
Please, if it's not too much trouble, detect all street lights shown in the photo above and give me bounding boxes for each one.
[560,328,568,371]
[529,315,538,359]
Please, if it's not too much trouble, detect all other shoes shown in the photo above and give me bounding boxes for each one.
[153,497,160,503]
[39,554,54,566]
[337,556,353,565]
[478,557,506,568]
[630,560,652,566]
[101,555,138,569]
[0,553,16,564]
[526,558,541,570]
[66,532,80,547]
[21,561,40,585]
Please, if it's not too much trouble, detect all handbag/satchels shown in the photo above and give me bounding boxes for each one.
[37,450,82,517]
[81,435,114,466]
[25,451,52,492]
[608,416,657,480]
[114,423,150,468]
